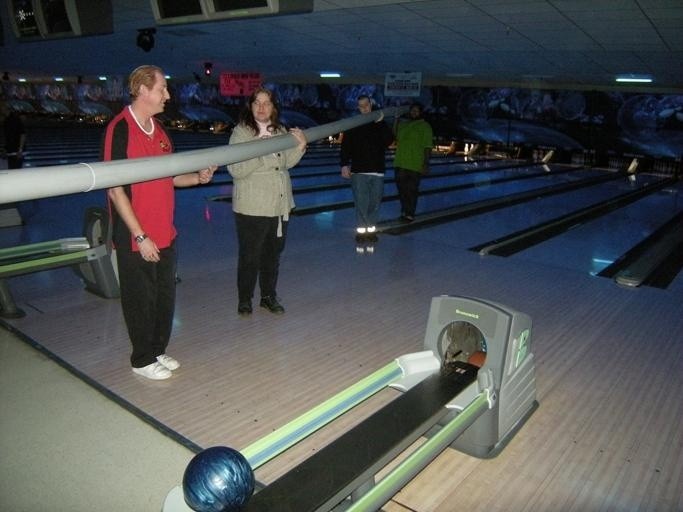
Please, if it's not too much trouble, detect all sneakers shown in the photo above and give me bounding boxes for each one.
[133,352,179,380]
[260,295,284,314]
[356,226,379,244]
[398,216,413,224]
[238,299,252,315]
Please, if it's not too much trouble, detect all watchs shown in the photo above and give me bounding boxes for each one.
[133,233,148,243]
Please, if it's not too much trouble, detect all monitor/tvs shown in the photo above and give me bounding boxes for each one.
[205,0,312,20]
[150,0,208,25]
[34,0,114,40]
[7,0,44,44]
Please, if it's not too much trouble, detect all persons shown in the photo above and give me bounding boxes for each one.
[340,94,396,242]
[226,88,307,316]
[392,103,434,223]
[102,64,219,380]
